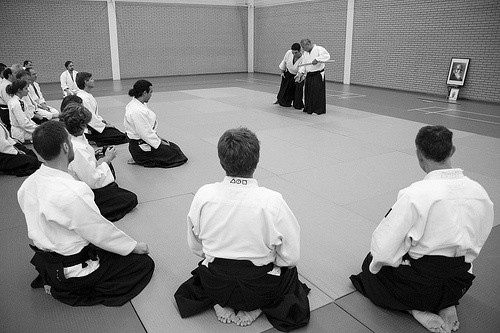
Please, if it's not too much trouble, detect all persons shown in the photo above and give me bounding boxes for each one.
[58,103,138,222]
[124,79,188,168]
[349,125,495,333]
[274,37,330,115]
[0,60,84,177]
[174,127,312,332]
[16,121,155,306]
[75,71,129,147]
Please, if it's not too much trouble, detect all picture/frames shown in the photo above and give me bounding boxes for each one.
[445,57,470,86]
[449,88,460,100]
